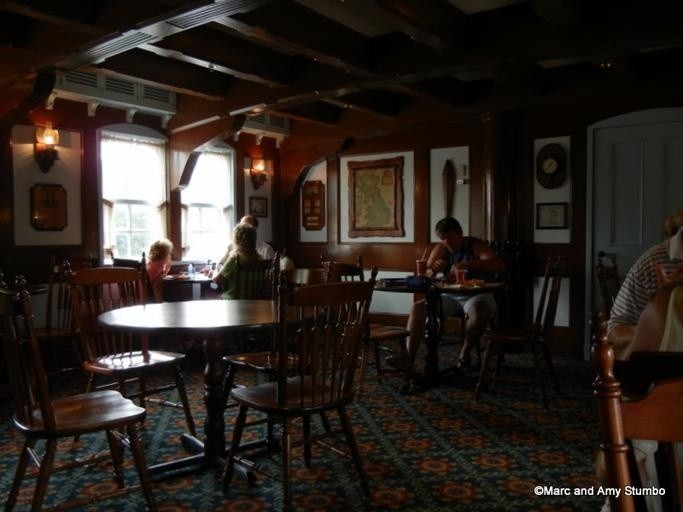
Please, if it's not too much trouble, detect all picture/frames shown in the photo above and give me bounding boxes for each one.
[347,155,406,238]
[28,182,69,232]
[301,181,325,231]
[249,196,269,218]
[535,202,568,230]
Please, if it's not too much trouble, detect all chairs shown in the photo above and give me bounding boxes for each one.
[585,247,683,511]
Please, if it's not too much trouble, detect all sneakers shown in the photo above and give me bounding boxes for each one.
[385,353,409,367]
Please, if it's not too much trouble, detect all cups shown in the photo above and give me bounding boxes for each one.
[416,259,427,277]
[456,270,468,283]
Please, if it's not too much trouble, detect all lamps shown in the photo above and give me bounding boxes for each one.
[250,158,267,191]
[32,123,60,174]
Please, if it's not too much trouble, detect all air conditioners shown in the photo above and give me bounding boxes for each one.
[234,112,292,150]
[47,68,178,132]
[69,2,266,68]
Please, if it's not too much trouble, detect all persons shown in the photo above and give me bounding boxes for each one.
[598,279,683,512]
[384,215,505,382]
[146,242,173,306]
[604,207,682,349]
[218,214,275,278]
[215,224,265,300]
